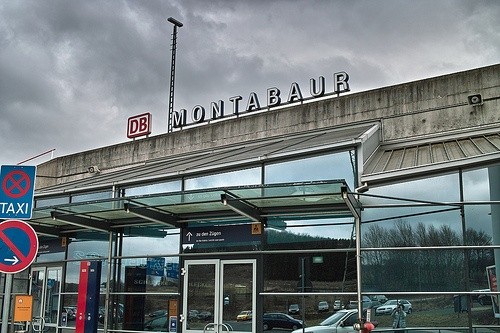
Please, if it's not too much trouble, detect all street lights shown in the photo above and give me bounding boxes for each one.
[166,17,184,133]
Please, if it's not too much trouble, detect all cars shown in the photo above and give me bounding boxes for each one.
[376,300,413,316]
[263,313,307,332]
[236,311,252,322]
[471,289,494,306]
[62,303,212,333]
[289,304,300,315]
[317,301,329,311]
[333,300,345,311]
[345,295,388,310]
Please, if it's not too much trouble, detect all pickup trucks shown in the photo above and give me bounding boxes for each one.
[291,309,458,333]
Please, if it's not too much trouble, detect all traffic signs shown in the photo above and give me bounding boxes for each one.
[182,222,262,244]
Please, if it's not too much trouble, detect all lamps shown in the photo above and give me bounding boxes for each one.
[124,199,181,228]
[124,226,167,238]
[340,186,361,219]
[50,208,112,233]
[30,224,61,237]
[220,189,261,222]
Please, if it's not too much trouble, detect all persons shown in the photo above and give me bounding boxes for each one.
[390,304,407,333]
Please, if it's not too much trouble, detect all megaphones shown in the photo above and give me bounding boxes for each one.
[467,94,484,106]
[88,166,98,174]
